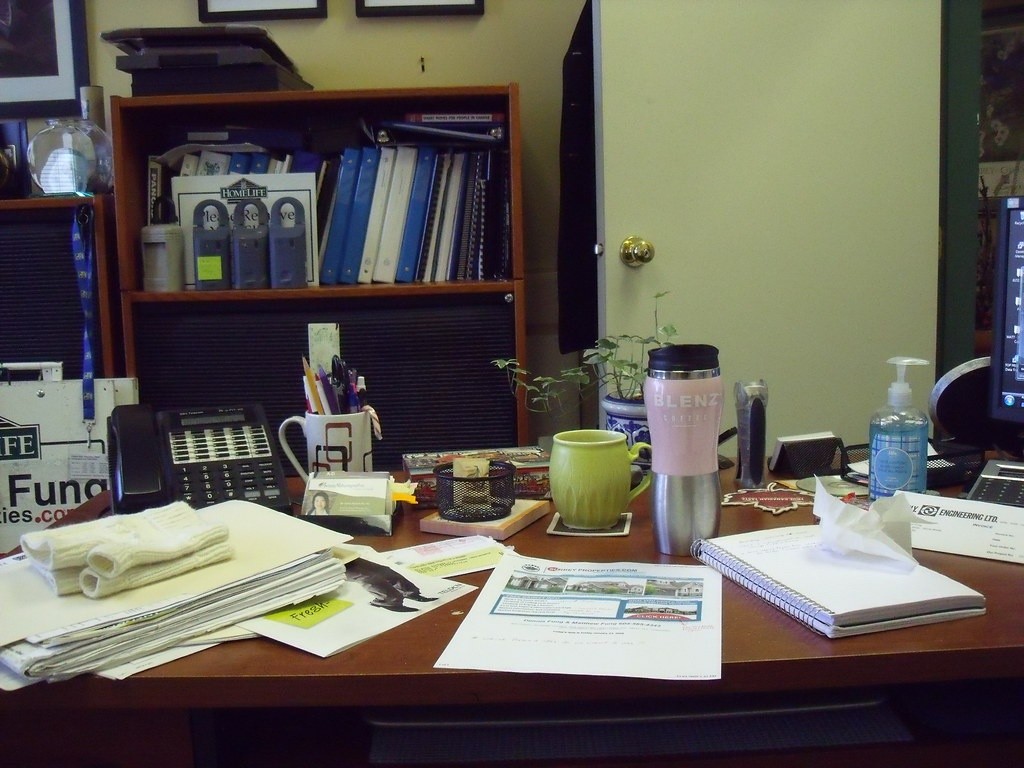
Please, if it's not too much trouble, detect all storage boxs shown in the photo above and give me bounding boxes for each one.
[403,444,552,508]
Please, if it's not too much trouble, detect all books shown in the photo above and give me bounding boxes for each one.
[690,524,985,637]
[100,24,511,286]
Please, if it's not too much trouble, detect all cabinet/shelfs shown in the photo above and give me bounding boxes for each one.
[0,192,114,385]
[109,81,529,475]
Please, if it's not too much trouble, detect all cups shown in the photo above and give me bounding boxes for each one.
[278,410,372,485]
[548,429,653,530]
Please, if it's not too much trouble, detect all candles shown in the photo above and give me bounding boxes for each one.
[453,457,490,506]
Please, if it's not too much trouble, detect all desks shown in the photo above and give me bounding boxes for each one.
[0,444,1024,768]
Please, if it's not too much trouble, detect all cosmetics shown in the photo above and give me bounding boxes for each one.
[868,357,929,503]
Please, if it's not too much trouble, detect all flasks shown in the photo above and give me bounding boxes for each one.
[644,344,723,555]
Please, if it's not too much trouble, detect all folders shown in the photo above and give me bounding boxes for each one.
[321,141,432,284]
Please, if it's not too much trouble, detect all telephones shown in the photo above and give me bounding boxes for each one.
[104,399,295,518]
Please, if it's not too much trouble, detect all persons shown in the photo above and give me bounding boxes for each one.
[305,491,330,515]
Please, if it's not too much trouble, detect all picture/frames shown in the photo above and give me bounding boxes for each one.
[0,0,91,119]
[197,0,328,23]
[355,0,485,17]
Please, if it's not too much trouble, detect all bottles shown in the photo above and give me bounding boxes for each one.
[139,193,184,291]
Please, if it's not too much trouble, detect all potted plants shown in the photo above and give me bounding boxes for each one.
[490,291,677,471]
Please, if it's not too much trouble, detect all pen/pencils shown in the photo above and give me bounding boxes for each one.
[301,352,368,414]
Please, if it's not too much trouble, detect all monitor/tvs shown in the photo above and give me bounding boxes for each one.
[991,195,1024,424]
